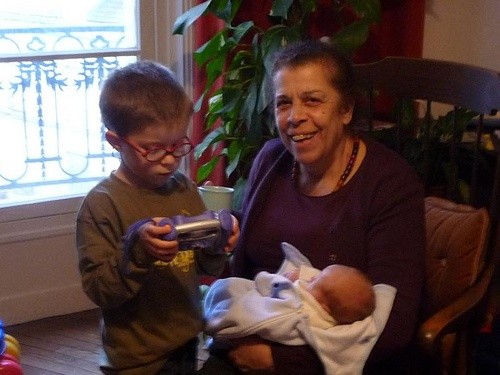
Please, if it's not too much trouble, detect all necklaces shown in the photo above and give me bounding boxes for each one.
[286,130,359,195]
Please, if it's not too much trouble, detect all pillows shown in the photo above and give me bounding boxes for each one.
[420,195,490,326]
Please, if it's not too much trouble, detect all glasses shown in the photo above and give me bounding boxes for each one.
[117,133,194,164]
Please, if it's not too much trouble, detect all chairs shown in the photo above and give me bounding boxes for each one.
[345,56,500,375]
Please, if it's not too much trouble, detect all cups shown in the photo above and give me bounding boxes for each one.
[197,184,235,212]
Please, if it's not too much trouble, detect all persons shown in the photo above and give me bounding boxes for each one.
[75,60,241,375]
[198,42,423,374]
[200,263,380,345]
